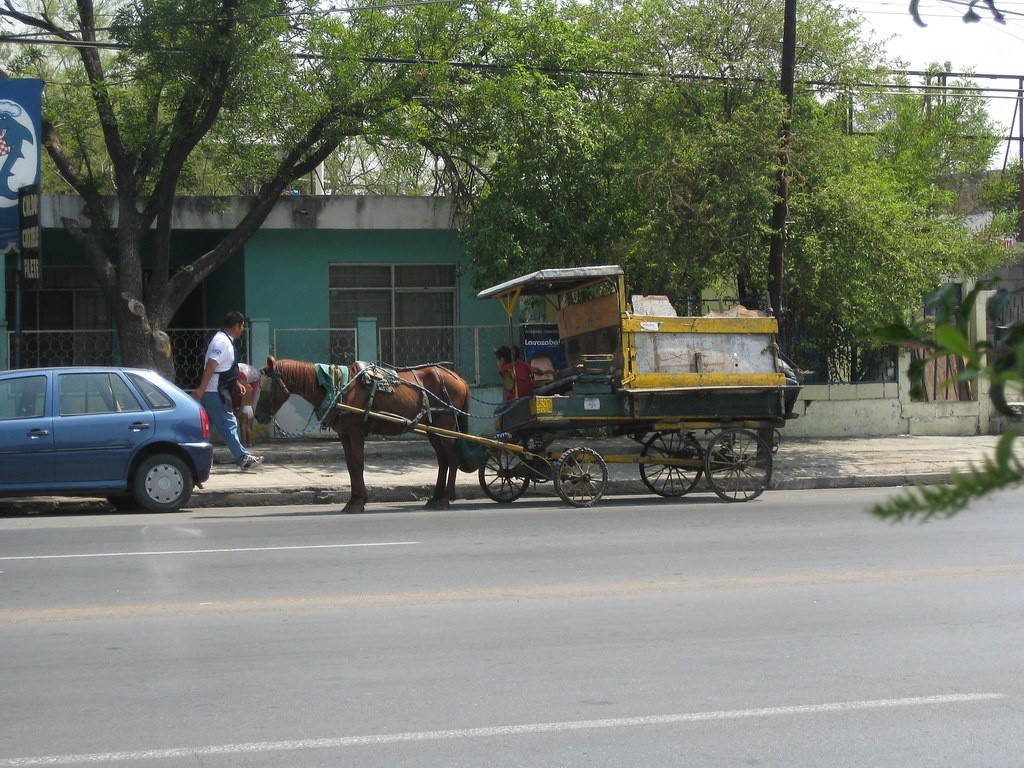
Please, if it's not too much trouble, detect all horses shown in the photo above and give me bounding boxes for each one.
[252,354,470,514]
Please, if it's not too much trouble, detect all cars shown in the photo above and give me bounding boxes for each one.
[0,366,213,513]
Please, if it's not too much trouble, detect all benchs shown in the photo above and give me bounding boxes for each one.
[535,352,616,395]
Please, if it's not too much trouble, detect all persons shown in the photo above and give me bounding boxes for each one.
[528,352,557,381]
[232,362,260,447]
[192,312,265,470]
[493,344,535,414]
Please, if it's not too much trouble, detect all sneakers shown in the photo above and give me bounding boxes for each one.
[241,455,264,472]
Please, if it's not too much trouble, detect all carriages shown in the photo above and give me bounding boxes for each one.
[253,264,813,515]
